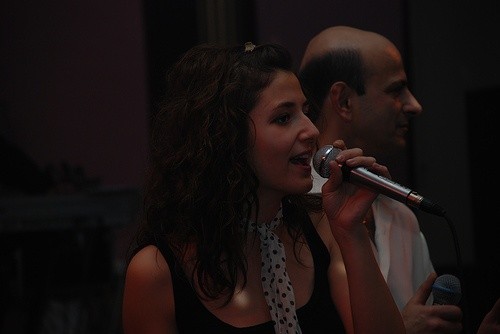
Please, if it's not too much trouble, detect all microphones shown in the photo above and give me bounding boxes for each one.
[313,145,447,217]
[431,274,461,305]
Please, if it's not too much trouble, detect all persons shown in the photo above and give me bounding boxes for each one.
[297,25,500,334]
[121,39,408,334]
